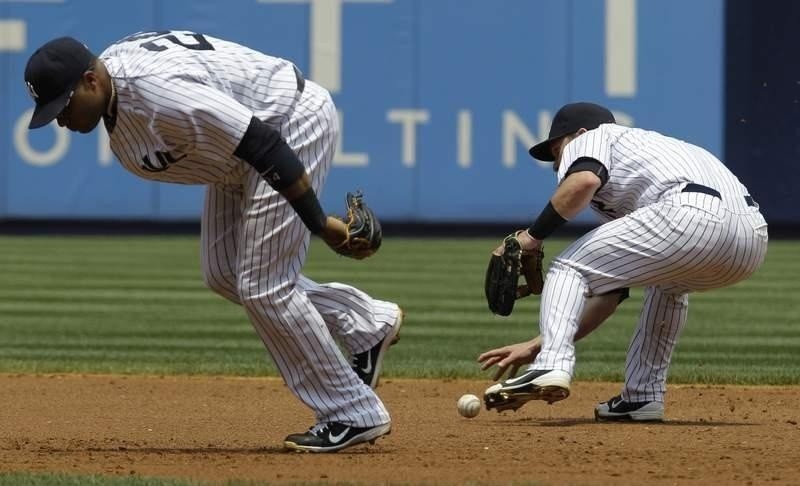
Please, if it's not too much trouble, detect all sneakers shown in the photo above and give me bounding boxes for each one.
[283,416,392,455]
[349,306,402,389]
[484,370,572,412]
[594,394,666,423]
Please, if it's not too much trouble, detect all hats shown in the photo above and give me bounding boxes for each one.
[25,37,85,130]
[529,102,616,162]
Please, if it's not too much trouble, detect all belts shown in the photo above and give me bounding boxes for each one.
[682,182,754,207]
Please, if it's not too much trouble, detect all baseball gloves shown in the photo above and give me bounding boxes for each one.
[335,191,382,258]
[486,229,545,316]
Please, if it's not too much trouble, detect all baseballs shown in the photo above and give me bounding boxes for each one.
[457,392,481,418]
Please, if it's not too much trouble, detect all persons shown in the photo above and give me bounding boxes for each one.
[478,102,769,422]
[24,29,405,453]
